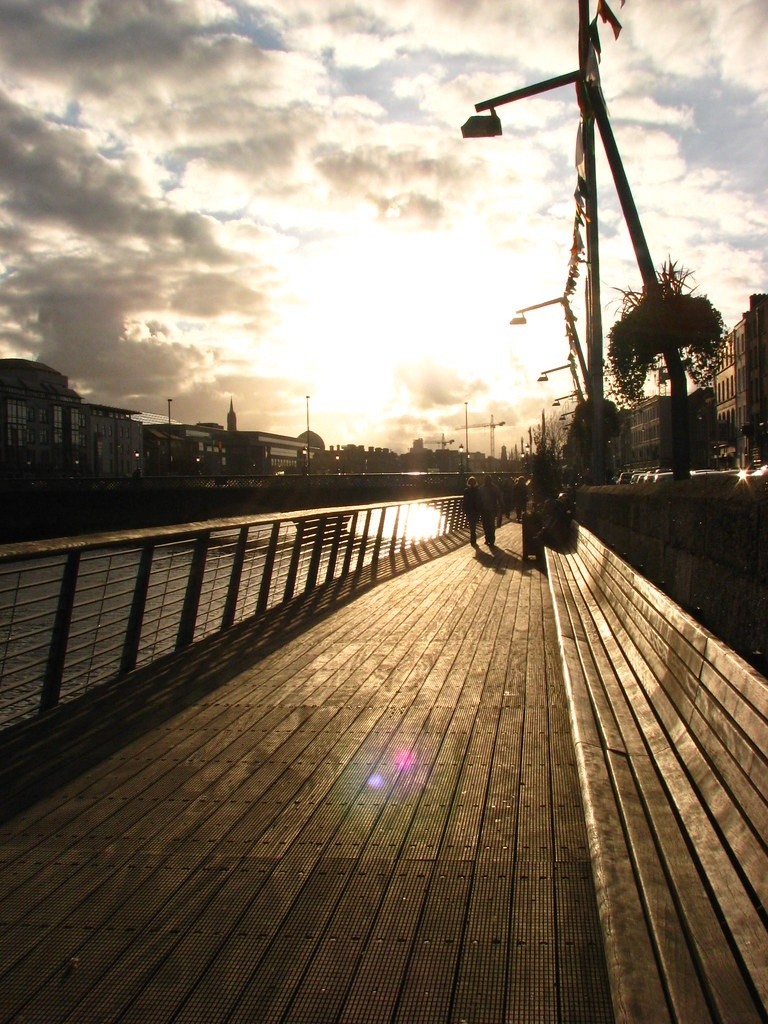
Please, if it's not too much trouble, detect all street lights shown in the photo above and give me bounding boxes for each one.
[537,353,587,404]
[464,402,469,471]
[306,395,311,475]
[510,297,592,392]
[167,398,172,474]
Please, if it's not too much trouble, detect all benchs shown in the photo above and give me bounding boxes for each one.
[545,519,768,1024]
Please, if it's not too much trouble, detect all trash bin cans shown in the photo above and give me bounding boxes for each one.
[522,513,543,560]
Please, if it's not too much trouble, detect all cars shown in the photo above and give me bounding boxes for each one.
[612,464,768,486]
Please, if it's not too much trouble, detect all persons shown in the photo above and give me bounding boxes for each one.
[462,473,574,567]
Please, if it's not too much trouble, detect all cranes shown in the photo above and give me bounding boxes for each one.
[456,413,506,472]
[426,432,454,449]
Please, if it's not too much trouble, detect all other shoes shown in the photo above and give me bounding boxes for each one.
[490,542,494,548]
[484,537,490,544]
[471,539,476,547]
